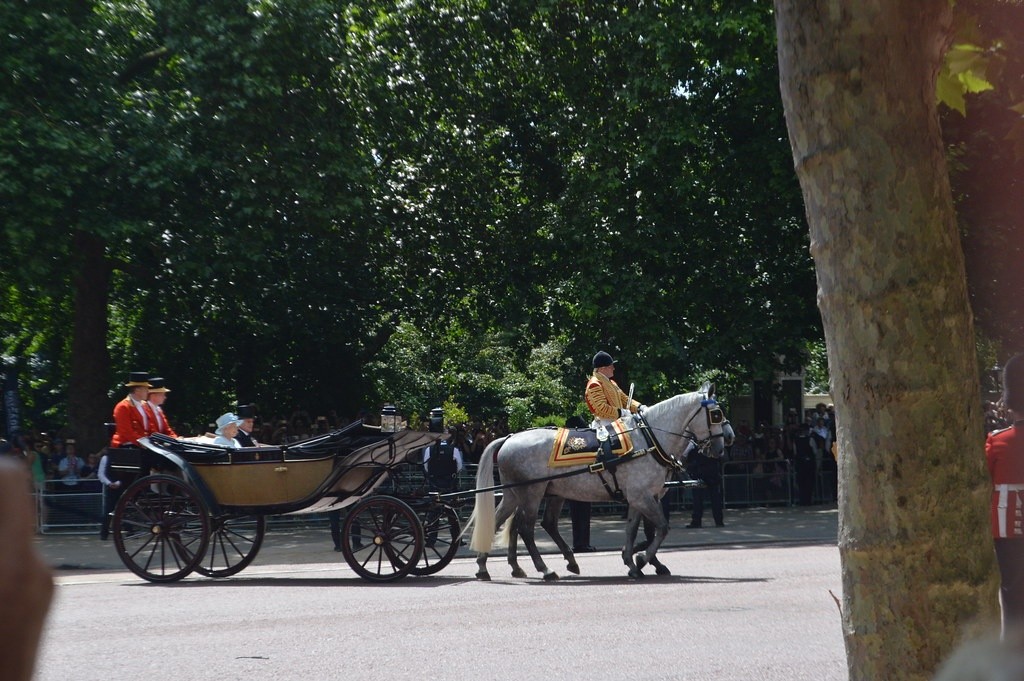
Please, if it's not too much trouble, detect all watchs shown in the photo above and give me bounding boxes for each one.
[637,404,642,413]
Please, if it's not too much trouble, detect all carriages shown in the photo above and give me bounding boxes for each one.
[108,379,735,581]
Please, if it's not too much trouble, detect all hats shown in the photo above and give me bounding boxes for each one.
[215,412,244,435]
[124,372,153,386]
[1004,353,1024,410]
[148,378,171,392]
[736,425,750,439]
[236,405,264,420]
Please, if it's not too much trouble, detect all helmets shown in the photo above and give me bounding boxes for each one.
[592,351,619,368]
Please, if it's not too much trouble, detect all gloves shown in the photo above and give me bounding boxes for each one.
[620,409,631,417]
[639,404,647,411]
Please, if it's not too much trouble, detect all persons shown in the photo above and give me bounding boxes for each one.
[584,349,651,467]
[329,501,365,552]
[424,429,467,547]
[644,497,670,540]
[686,446,725,528]
[669,403,841,511]
[983,398,1014,439]
[168,398,339,450]
[982,352,1024,660]
[449,423,500,465]
[0,432,139,541]
[146,376,178,441]
[569,499,597,552]
[109,371,174,496]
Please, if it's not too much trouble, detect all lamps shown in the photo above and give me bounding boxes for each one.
[381,403,401,459]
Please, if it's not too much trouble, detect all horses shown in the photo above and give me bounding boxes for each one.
[466,380,735,581]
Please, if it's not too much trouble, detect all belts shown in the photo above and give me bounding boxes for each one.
[994,483,1024,491]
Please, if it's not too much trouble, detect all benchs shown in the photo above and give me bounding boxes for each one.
[104,423,141,467]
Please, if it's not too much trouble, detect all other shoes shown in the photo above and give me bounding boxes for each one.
[334,545,343,552]
[353,544,364,550]
[425,540,434,547]
[460,540,467,547]
[716,520,724,527]
[686,522,701,528]
